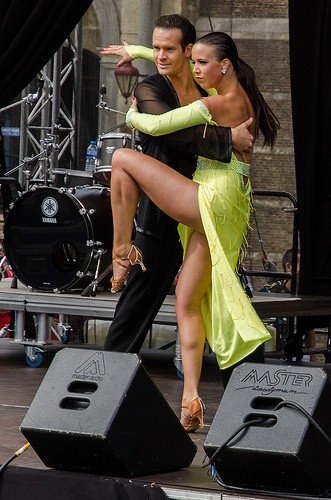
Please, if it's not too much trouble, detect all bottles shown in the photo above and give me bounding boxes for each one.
[85,140,98,173]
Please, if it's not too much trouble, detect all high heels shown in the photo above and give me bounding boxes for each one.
[180,397,206,432]
[111,245,146,294]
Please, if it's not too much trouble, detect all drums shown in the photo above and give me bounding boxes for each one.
[92,132,143,187]
[3,184,139,294]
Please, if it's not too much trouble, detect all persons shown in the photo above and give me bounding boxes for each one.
[100,31,281,432]
[269,248,320,362]
[102,14,266,390]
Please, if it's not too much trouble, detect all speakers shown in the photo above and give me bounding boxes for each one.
[30,45,99,170]
[18,349,197,474]
[202,362,330,495]
